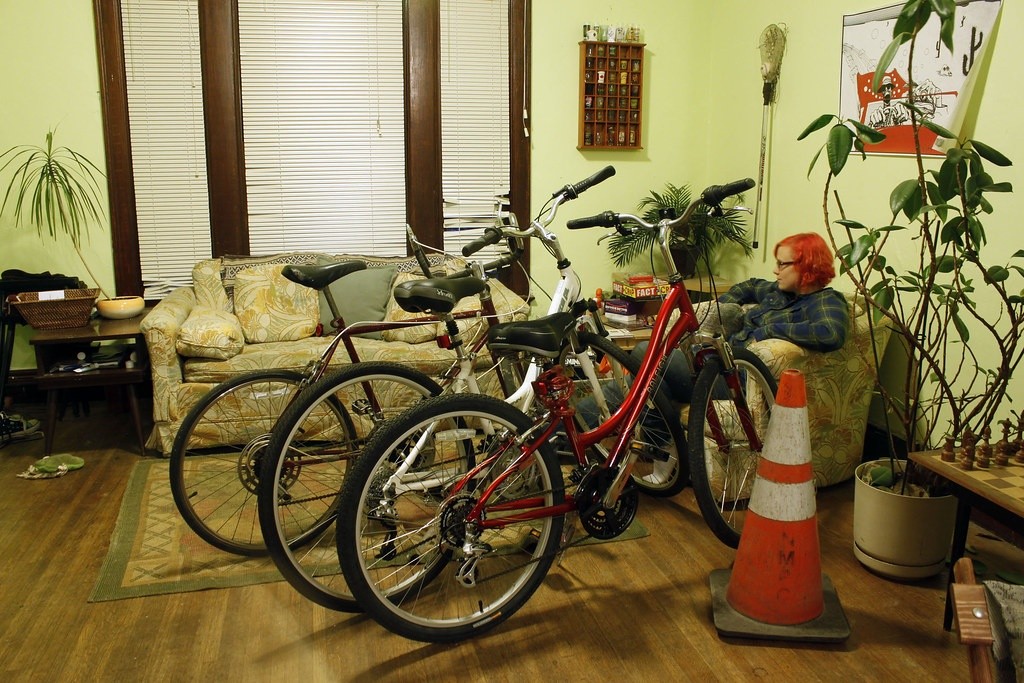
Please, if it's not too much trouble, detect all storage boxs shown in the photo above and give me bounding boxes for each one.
[612,282,671,298]
[604,300,637,315]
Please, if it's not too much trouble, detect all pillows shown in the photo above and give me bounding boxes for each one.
[438,295,489,347]
[316,255,398,341]
[381,272,438,344]
[175,304,245,361]
[233,263,321,343]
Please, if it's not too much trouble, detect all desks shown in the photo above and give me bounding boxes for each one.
[908,443,1024,632]
[0,277,91,419]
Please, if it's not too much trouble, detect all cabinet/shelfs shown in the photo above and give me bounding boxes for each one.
[576,40,647,151]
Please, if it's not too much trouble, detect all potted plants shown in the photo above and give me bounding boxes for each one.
[0,121,146,319]
[795,0,1024,584]
[608,179,756,275]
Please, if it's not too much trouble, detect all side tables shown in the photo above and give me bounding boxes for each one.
[28,311,150,458]
[601,315,653,351]
[653,271,736,300]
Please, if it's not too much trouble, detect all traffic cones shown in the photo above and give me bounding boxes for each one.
[710,369,855,646]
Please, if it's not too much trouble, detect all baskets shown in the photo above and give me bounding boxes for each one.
[6,288,101,330]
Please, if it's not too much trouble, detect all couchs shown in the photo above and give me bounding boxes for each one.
[653,290,895,502]
[140,253,531,458]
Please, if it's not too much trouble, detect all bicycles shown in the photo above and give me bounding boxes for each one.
[346,180,780,640]
[169,164,692,617]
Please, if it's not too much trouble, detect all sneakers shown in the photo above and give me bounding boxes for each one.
[0,411,44,442]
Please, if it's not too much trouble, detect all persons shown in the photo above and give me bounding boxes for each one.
[553,233,850,446]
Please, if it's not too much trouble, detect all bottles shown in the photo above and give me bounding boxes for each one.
[583,24,640,146]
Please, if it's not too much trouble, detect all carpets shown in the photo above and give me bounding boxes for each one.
[87,434,652,603]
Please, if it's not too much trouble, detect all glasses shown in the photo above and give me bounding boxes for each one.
[777,260,793,269]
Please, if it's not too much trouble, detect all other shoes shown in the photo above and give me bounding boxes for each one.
[643,442,679,485]
